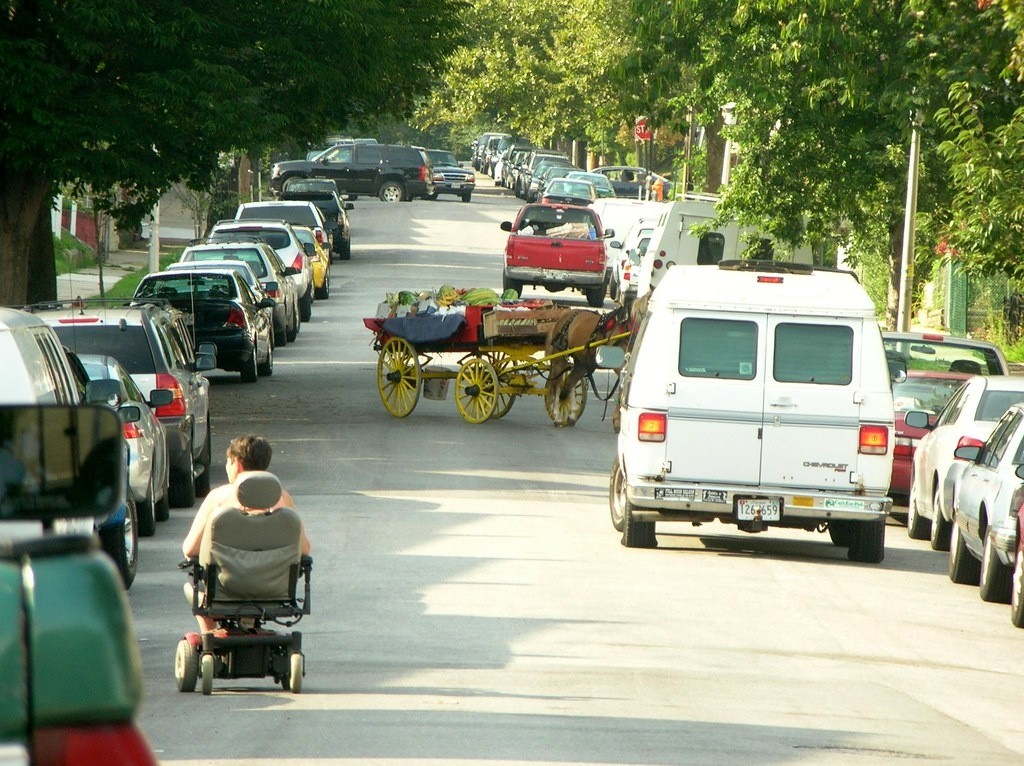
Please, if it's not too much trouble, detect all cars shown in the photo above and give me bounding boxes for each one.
[169,131,675,352]
[904,374,1024,551]
[1011,466,1024,631]
[888,368,981,500]
[75,353,173,536]
[948,402,1024,605]
[121,269,276,383]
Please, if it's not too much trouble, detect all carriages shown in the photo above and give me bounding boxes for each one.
[362,283,657,434]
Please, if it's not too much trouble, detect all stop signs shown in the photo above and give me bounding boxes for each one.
[635,118,652,141]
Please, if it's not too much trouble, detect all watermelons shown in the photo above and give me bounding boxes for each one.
[398,290,414,305]
[464,289,519,305]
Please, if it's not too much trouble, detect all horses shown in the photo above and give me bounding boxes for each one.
[546,283,656,429]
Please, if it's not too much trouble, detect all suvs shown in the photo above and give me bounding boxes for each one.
[268,144,434,202]
[25,295,216,509]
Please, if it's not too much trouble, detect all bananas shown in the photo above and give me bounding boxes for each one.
[438,289,461,305]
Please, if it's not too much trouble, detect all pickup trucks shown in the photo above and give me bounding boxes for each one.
[501,203,616,307]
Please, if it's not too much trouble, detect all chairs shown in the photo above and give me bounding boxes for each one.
[246,261,263,276]
[578,188,589,196]
[627,172,635,180]
[948,359,982,377]
[886,350,905,367]
[158,287,177,302]
[265,234,286,250]
[896,396,925,409]
[555,183,564,195]
[986,394,1012,418]
[211,284,229,299]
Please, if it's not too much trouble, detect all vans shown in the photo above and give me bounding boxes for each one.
[882,331,1011,382]
[594,258,908,564]
[608,195,819,325]
[0,306,139,592]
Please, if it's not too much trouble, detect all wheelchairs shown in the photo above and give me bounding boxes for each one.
[174,471,312,695]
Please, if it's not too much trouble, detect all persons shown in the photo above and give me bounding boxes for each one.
[182,435,312,640]
